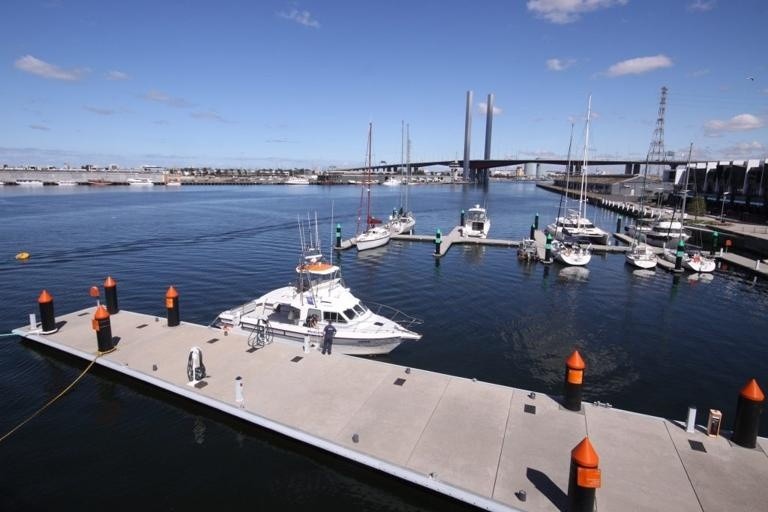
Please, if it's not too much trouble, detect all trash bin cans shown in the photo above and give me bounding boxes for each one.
[706,409,723,437]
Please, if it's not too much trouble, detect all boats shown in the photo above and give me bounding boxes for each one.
[467,202,490,237]
[551,240,591,265]
[208,198,424,357]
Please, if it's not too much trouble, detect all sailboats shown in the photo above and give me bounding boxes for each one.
[624,142,716,273]
[352,122,416,251]
[546,91,611,244]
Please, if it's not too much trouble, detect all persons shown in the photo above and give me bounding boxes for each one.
[321,320,336,355]
